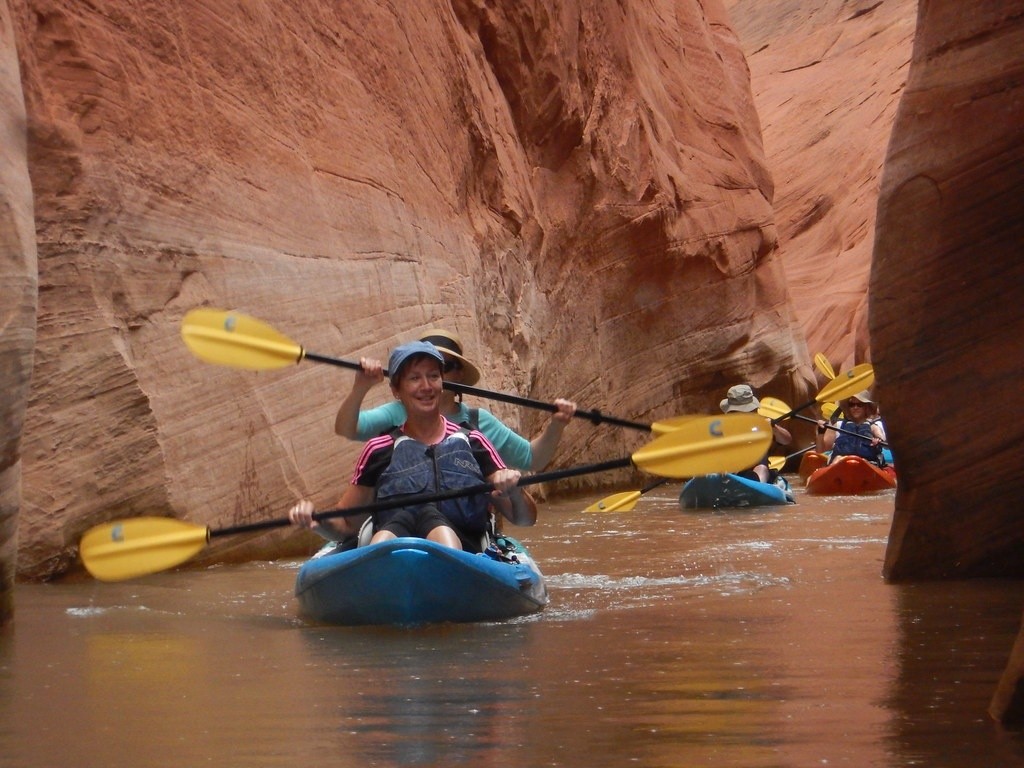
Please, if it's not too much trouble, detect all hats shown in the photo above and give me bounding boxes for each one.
[838,388,877,417]
[388,340,445,382]
[418,329,480,387]
[720,384,759,414]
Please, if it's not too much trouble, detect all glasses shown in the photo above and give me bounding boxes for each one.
[442,358,463,372]
[848,402,869,408]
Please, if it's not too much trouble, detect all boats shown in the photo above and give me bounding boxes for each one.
[677,473,798,510]
[797,449,828,484]
[292,524,547,621]
[806,455,897,496]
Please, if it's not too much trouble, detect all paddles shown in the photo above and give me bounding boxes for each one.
[76,406,773,586]
[816,400,847,421]
[179,299,712,446]
[576,359,879,517]
[755,393,889,449]
[765,444,814,473]
[812,349,840,379]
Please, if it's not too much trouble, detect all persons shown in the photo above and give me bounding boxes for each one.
[719,385,791,483]
[287,341,537,552]
[334,329,576,472]
[816,390,887,468]
